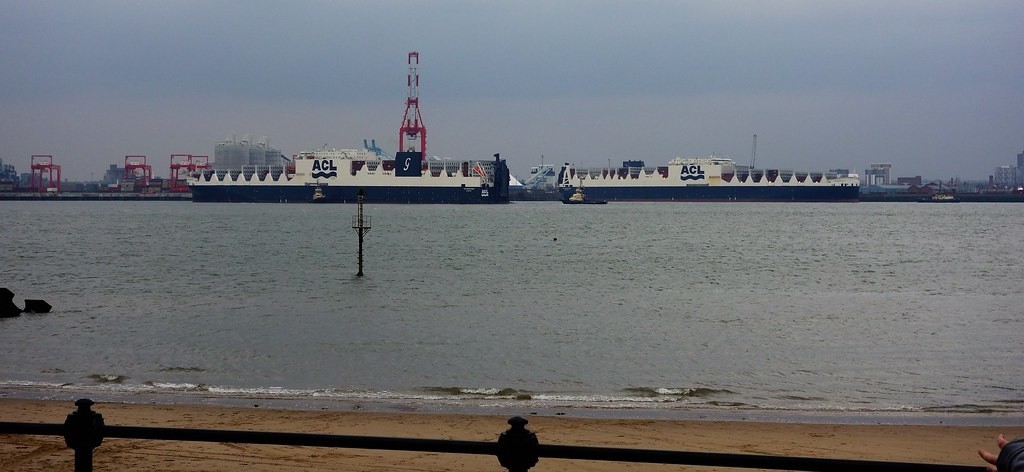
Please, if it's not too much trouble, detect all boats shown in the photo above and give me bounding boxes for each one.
[913,194,961,203]
[312,187,327,203]
[561,186,608,204]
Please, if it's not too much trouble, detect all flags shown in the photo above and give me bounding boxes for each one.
[474,164,486,177]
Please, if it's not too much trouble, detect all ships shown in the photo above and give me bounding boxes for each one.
[187,146,525,205]
[557,156,861,203]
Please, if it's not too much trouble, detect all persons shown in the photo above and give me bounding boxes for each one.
[978,434,1024,472]
